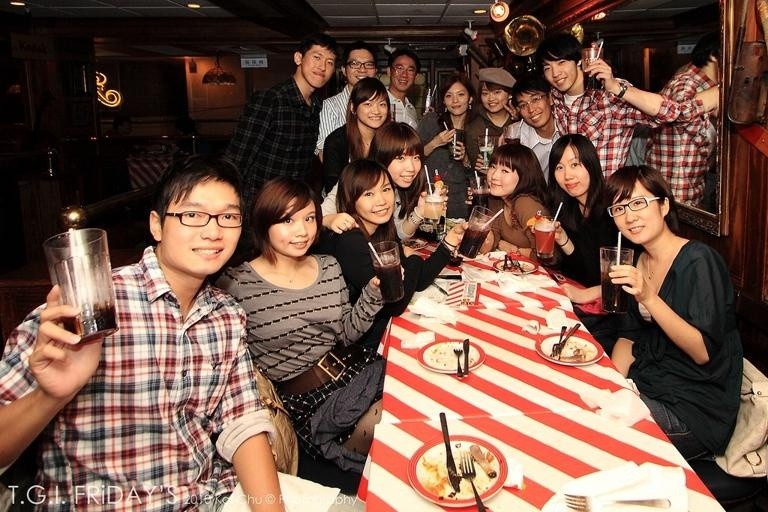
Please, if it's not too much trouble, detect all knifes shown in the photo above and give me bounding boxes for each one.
[461,336,471,376]
[546,323,587,356]
[439,411,461,496]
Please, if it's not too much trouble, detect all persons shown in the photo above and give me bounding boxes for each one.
[1,151,287,512]
[214,176,406,473]
[603,165,743,463]
[321,161,465,316]
[528,133,643,359]
[643,33,720,208]
[0,32,681,271]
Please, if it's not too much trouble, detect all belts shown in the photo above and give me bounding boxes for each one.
[278,351,350,395]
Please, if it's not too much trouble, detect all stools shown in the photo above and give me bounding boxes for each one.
[686,453,760,512]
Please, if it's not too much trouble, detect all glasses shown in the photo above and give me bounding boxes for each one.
[519,94,547,109]
[607,197,663,218]
[392,65,419,74]
[343,61,375,70]
[164,211,242,228]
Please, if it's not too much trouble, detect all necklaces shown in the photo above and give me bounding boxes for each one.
[642,247,660,282]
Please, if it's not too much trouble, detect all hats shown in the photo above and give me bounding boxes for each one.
[479,68,516,89]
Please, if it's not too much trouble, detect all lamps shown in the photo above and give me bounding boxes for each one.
[590,13,607,22]
[463,19,478,41]
[383,37,397,55]
[486,0,509,21]
[202,53,237,87]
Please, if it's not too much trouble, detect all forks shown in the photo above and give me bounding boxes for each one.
[459,457,488,507]
[564,492,675,512]
[551,326,566,363]
[453,346,462,376]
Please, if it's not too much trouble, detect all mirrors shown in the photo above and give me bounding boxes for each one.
[556,0,729,239]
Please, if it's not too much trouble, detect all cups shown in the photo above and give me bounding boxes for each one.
[503,126,521,145]
[478,135,495,169]
[469,176,490,215]
[422,88,437,116]
[458,205,496,259]
[44,228,120,342]
[599,245,634,314]
[370,240,405,303]
[424,180,448,225]
[447,128,464,159]
[534,214,556,259]
[581,47,605,90]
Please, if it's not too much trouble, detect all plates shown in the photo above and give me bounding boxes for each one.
[401,237,430,252]
[405,436,507,507]
[417,337,488,377]
[536,331,604,369]
[492,255,539,276]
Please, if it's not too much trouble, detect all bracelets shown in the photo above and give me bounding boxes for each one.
[560,235,570,247]
